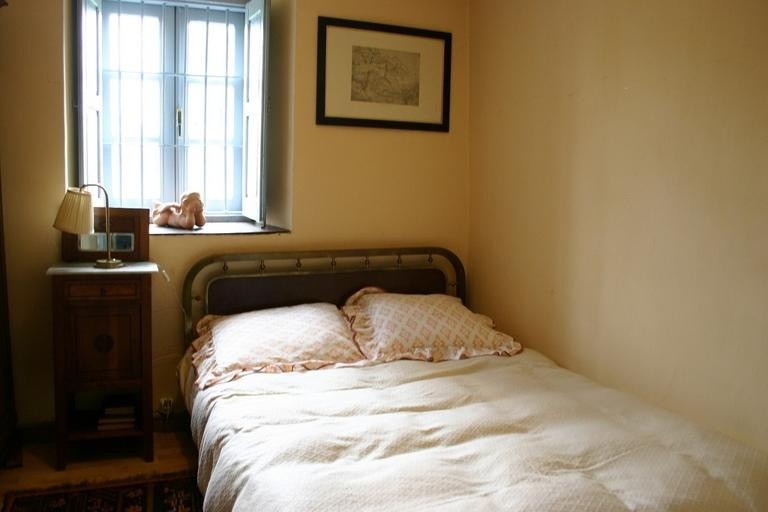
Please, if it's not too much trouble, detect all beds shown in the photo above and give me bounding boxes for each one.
[173,243,768,511]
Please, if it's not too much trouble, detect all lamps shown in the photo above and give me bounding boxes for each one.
[48,181,128,270]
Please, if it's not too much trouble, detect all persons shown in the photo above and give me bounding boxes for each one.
[149,190,207,229]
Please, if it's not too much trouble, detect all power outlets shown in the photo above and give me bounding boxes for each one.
[157,396,173,416]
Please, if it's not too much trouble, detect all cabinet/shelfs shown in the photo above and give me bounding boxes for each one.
[41,260,163,464]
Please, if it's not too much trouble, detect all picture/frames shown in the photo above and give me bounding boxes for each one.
[59,202,153,264]
[312,13,455,136]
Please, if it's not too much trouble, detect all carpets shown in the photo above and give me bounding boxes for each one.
[0,464,208,512]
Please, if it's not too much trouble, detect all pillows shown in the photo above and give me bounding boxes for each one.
[186,297,368,394]
[335,278,524,368]
